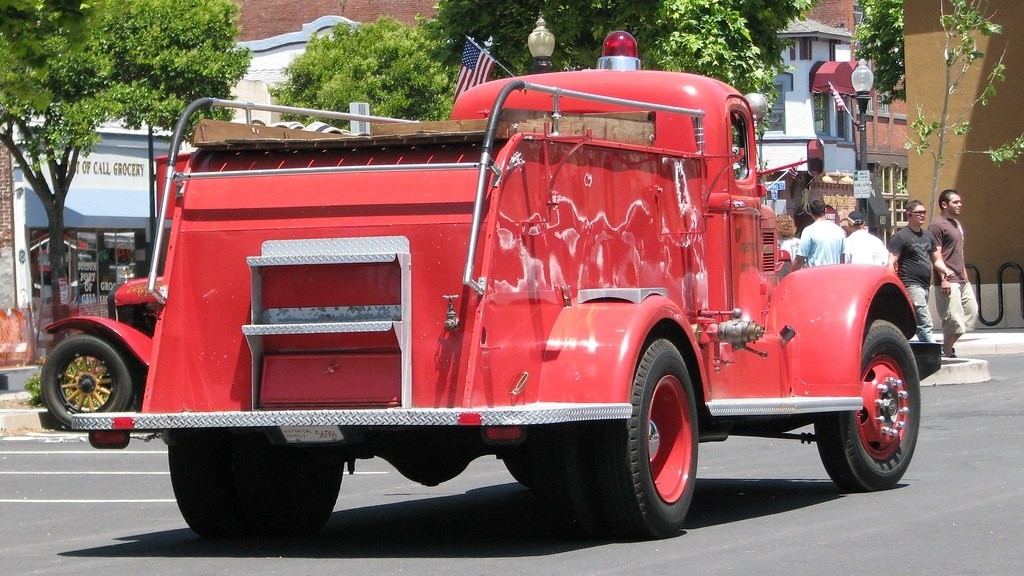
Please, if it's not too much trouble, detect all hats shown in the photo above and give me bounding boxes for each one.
[847,211,865,227]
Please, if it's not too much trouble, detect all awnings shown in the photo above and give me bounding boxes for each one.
[809,61,859,96]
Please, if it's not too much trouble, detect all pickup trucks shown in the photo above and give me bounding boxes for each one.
[40,30,944,538]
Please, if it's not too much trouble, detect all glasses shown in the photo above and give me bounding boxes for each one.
[911,211,926,216]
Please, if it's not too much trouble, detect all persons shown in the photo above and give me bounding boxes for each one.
[887,201,956,343]
[776,200,889,282]
[927,189,978,358]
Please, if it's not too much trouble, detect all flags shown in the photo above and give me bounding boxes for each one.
[453,36,495,105]
[828,80,846,111]
[788,160,802,179]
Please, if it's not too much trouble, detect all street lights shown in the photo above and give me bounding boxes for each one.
[851,59,876,229]
